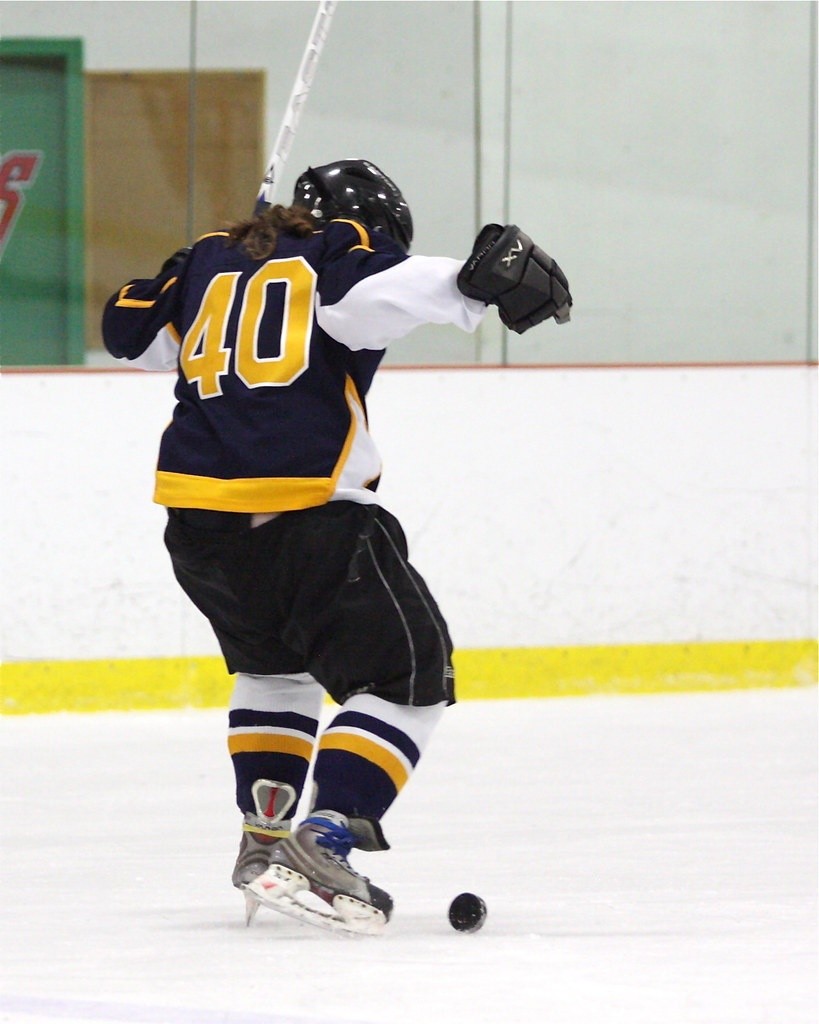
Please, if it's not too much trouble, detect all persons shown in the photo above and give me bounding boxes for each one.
[102,160,574,934]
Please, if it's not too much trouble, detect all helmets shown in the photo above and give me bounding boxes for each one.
[294,160,413,253]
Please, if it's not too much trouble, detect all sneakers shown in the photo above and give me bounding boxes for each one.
[232,779,291,928]
[240,781,394,937]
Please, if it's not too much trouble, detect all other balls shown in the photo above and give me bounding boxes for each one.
[449,892,487,934]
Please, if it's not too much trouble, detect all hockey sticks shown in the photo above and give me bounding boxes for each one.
[251,1,339,221]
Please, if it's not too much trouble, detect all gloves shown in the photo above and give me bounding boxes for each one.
[458,223,572,334]
